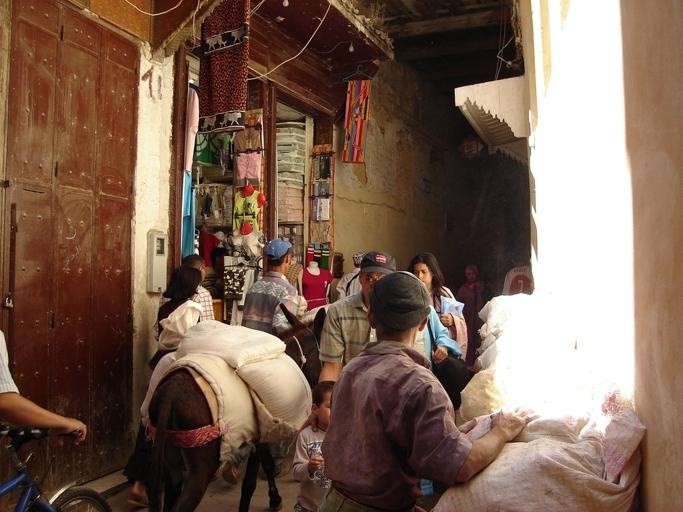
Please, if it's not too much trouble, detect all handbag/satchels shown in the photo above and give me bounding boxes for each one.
[434,347,476,410]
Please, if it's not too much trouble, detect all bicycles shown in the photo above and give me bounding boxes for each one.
[0,424,110,510]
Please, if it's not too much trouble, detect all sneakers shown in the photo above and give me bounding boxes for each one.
[222,461,240,483]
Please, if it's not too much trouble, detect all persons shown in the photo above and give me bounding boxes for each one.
[183,253,215,324]
[2,333,87,449]
[122,266,202,509]
[241,237,540,512]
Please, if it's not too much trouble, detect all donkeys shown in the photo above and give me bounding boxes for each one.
[137,303,328,512]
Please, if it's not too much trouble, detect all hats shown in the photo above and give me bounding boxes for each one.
[368,270,432,330]
[360,250,396,274]
[263,239,292,260]
[352,249,367,264]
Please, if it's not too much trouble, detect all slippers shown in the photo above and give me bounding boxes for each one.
[127,486,149,507]
